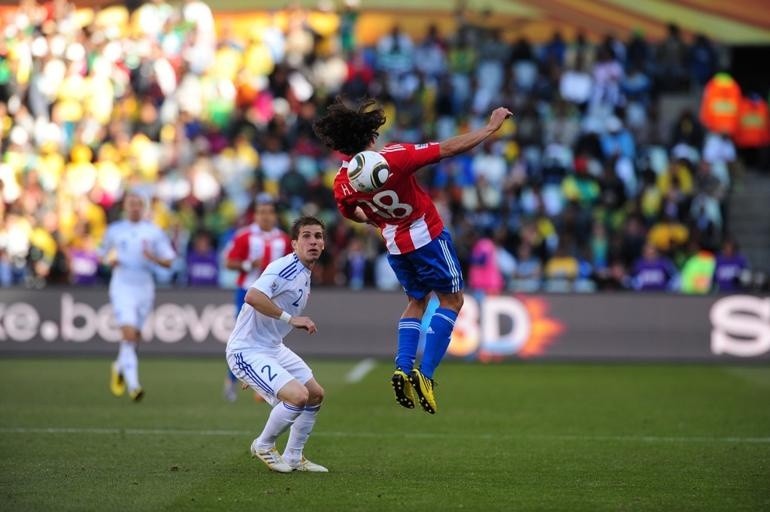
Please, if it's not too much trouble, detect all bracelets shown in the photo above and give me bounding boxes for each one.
[279,309,292,323]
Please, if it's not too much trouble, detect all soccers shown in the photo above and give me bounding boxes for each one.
[346,150,389,193]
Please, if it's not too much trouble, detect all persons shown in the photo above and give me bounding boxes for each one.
[224,216,334,474]
[308,95,514,414]
[2,0,770,298]
[220,194,293,405]
[91,191,176,404]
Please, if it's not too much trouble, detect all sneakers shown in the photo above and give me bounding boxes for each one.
[250,439,329,473]
[391,368,438,414]
[225,384,236,402]
[110,362,126,398]
[130,388,144,401]
